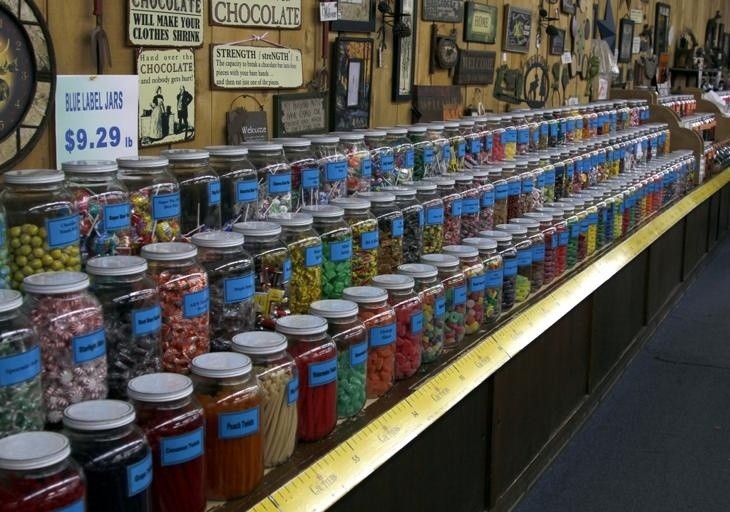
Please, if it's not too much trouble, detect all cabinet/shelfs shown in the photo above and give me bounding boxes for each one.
[2,113,730,512]
[611,86,730,182]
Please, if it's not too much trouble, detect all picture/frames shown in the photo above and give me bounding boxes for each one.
[561,0,577,15]
[550,29,566,55]
[331,36,374,132]
[273,93,330,135]
[392,2,417,101]
[330,1,377,32]
[654,3,671,55]
[503,4,533,53]
[465,1,498,45]
[618,18,635,62]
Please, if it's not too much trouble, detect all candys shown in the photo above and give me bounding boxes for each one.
[0,263,444,511]
[444,98,697,355]
[1,120,444,289]
[0,174,459,437]
[656,95,730,175]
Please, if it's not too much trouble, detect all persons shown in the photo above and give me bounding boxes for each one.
[150,85,165,141]
[176,85,193,129]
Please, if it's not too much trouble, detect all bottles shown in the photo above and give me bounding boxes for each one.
[396,263,445,362]
[245,143,293,217]
[534,111,548,148]
[516,152,544,211]
[497,223,532,302]
[381,186,425,264]
[162,149,223,234]
[86,256,163,400]
[494,155,517,219]
[275,136,320,211]
[549,200,577,268]
[302,204,353,300]
[453,171,480,240]
[505,158,534,217]
[525,110,540,151]
[354,127,395,189]
[190,352,267,500]
[23,271,108,426]
[501,116,517,158]
[437,120,466,171]
[356,191,404,276]
[442,245,484,335]
[342,286,396,399]
[329,196,380,285]
[468,163,494,234]
[4,167,80,299]
[479,230,518,311]
[332,132,372,194]
[659,93,697,117]
[271,211,323,315]
[470,117,493,165]
[119,152,181,245]
[1,432,85,511]
[232,221,292,334]
[0,289,42,443]
[420,252,466,347]
[140,241,210,375]
[372,274,422,379]
[705,140,730,182]
[64,158,133,263]
[510,218,545,293]
[405,178,444,255]
[231,330,301,467]
[420,122,452,175]
[567,149,698,266]
[483,161,507,225]
[275,314,338,442]
[544,98,649,148]
[126,372,208,511]
[431,175,462,245]
[206,145,260,223]
[538,206,567,273]
[311,298,369,418]
[681,113,717,144]
[0,189,13,291]
[511,112,530,155]
[192,229,256,352]
[403,124,433,175]
[462,238,502,322]
[487,116,503,160]
[61,399,153,511]
[381,127,414,186]
[301,134,349,203]
[458,120,481,168]
[540,122,672,204]
[523,212,555,283]
